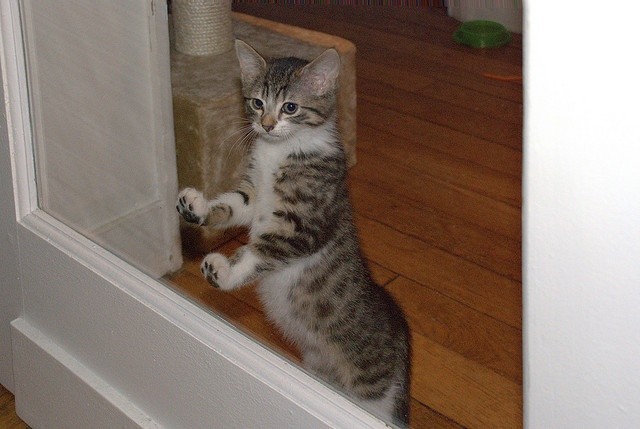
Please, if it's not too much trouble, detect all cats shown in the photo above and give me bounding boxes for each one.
[174,40,413,427]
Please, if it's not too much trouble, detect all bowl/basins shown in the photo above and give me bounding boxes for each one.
[452,19,512,50]
